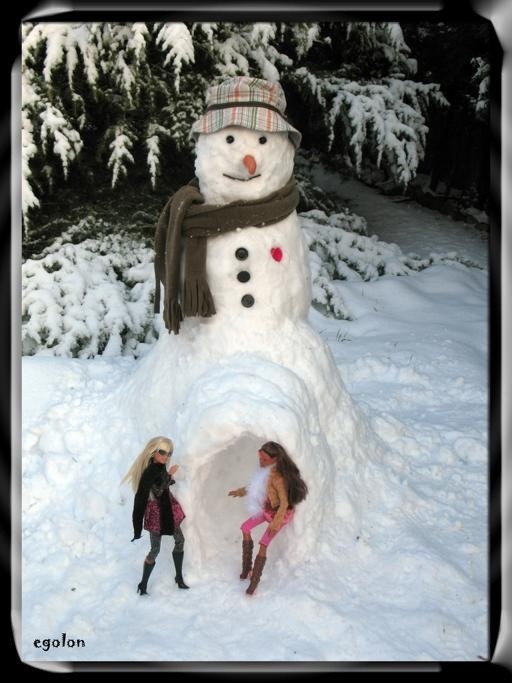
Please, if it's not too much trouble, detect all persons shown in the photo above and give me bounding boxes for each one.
[119,436,190,595]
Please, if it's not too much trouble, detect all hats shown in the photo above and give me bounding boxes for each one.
[191,76,302,147]
